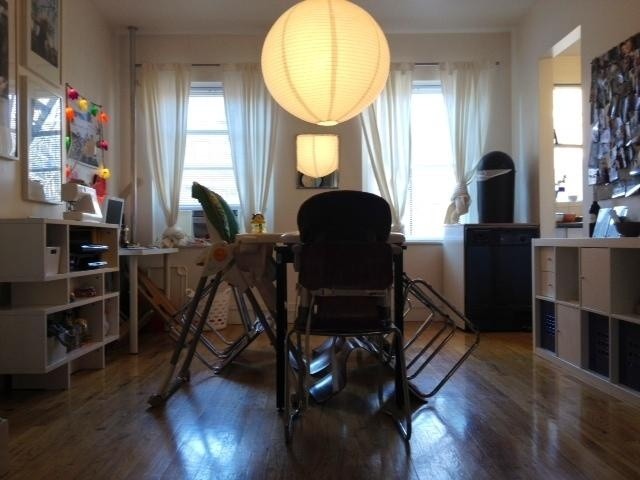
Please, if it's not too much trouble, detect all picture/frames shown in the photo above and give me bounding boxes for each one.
[1,0,65,207]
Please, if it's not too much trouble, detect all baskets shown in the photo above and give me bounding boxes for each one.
[185,287,231,330]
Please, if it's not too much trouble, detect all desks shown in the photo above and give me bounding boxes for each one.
[118,246,181,356]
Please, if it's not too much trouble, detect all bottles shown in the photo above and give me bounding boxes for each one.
[589,201,600,237]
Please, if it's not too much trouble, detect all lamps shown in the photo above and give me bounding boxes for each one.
[259,1,393,182]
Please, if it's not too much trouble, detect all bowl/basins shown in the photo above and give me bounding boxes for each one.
[568,196,577,202]
[576,216,583,222]
[614,222,640,237]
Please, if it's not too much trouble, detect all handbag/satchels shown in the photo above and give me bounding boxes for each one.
[47,316,76,346]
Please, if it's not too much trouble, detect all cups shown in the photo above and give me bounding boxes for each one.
[565,213,575,221]
[556,213,564,222]
[251,214,266,234]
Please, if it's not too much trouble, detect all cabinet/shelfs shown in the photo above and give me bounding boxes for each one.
[1,216,124,391]
[529,236,639,405]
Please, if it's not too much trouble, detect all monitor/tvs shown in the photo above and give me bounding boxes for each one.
[102,196,124,225]
